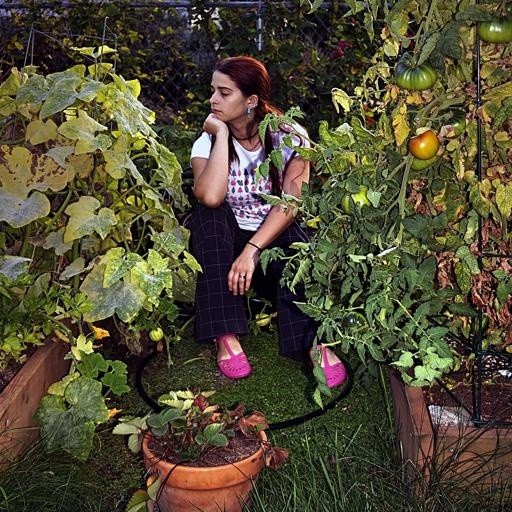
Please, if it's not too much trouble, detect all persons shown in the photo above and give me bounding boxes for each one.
[190,57,349,390]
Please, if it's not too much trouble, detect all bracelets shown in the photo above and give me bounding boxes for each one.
[248,242,261,251]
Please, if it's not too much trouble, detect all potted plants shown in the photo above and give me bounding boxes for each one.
[140,381,273,510]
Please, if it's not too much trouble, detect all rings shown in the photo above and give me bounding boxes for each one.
[239,276,245,279]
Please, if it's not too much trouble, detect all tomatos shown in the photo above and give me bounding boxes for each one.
[341,16,512,217]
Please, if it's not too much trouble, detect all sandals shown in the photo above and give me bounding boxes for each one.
[215,333,252,379]
[309,344,346,389]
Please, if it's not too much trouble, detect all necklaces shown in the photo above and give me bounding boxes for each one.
[231,128,259,140]
[247,138,261,152]
